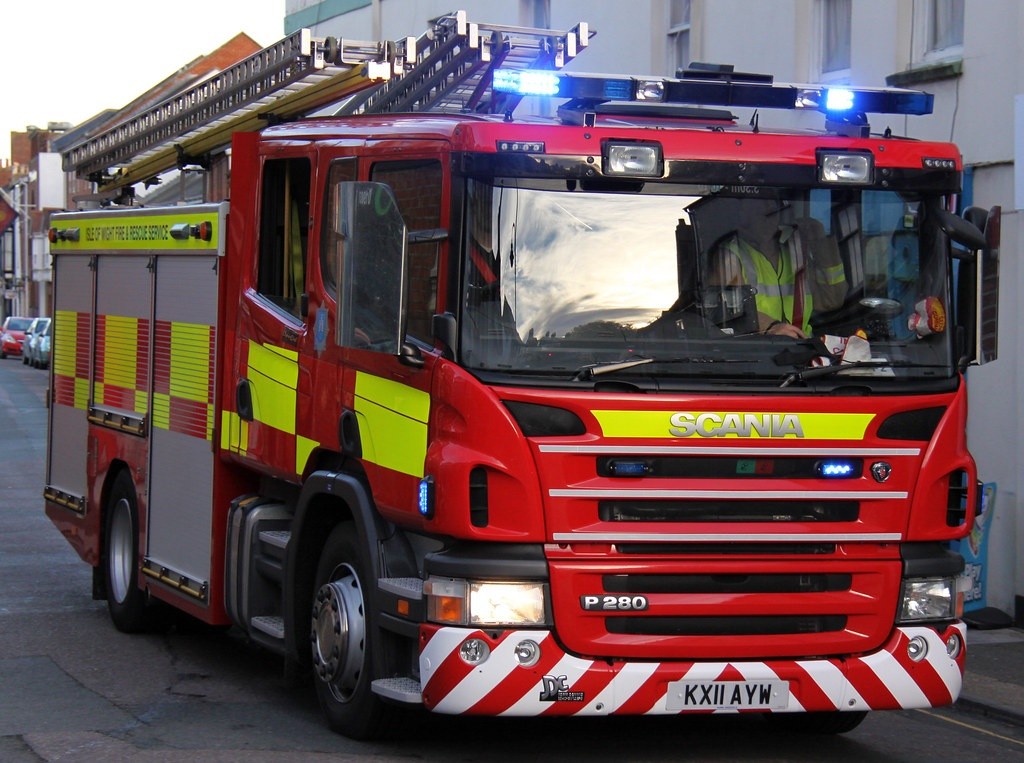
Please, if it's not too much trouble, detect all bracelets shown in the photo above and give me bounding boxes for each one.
[766,321,780,335]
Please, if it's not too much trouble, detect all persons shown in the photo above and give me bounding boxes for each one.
[425,189,534,312]
[709,185,848,342]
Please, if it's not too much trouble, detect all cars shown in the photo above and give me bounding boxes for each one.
[23,318,52,368]
[0,316,34,359]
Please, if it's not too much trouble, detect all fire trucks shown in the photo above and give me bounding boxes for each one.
[43,8,997,742]
